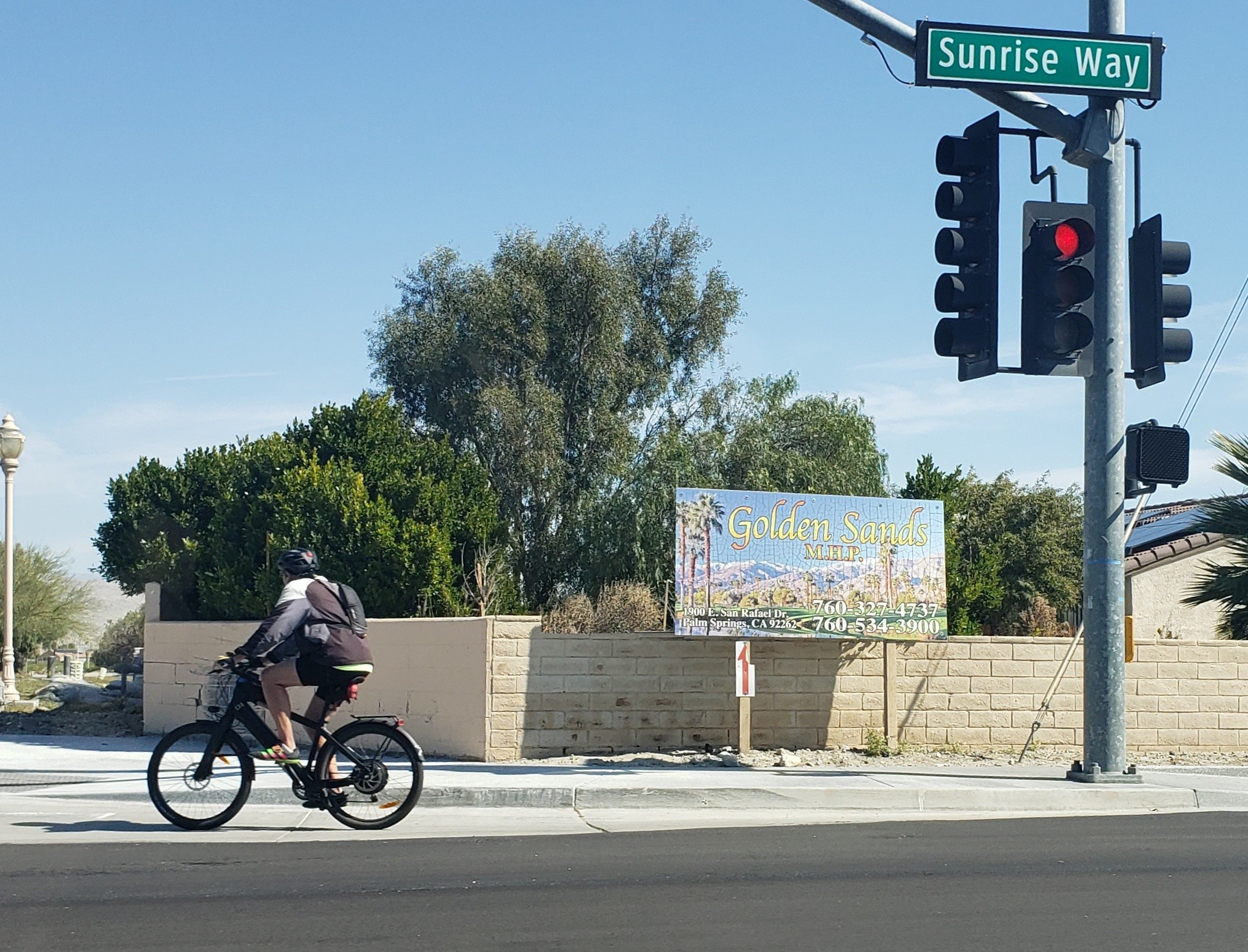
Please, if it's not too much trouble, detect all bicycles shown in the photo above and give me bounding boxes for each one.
[146,653,426,830]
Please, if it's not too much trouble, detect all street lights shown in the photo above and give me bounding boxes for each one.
[0,414,27,706]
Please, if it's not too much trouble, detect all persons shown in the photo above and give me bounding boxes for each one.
[217,548,374,807]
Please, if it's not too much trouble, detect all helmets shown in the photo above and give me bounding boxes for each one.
[277,547,320,576]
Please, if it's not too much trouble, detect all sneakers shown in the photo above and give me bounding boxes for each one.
[303,791,348,808]
[246,741,300,764]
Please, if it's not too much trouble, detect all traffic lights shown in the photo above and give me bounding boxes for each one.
[1129,213,1194,391]
[1020,199,1099,380]
[1126,424,1191,487]
[933,110,1004,382]
